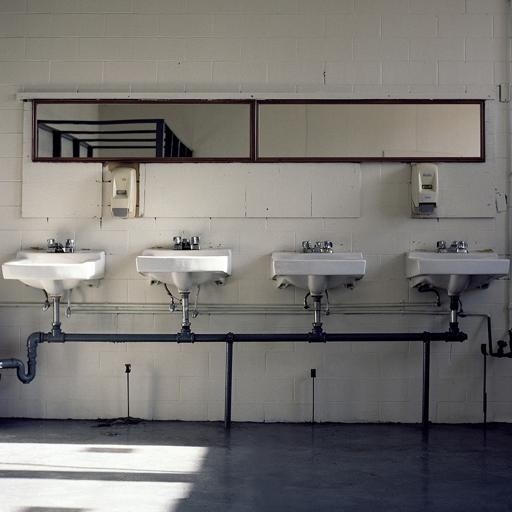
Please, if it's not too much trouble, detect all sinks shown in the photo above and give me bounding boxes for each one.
[135,249,232,274]
[270,252,367,280]
[406,250,510,278]
[2,251,106,280]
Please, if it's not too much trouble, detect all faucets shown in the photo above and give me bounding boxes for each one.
[450,242,465,249]
[313,242,323,252]
[182,239,191,250]
[49,242,66,252]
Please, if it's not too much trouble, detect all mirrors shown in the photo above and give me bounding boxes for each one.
[31,96,255,163]
[256,98,485,163]
